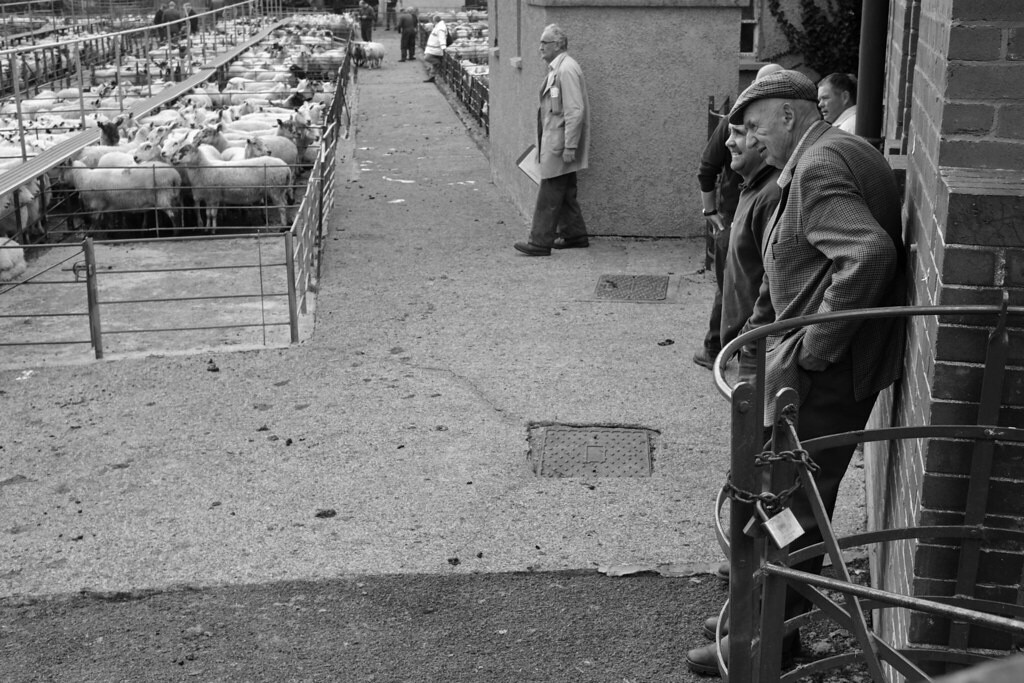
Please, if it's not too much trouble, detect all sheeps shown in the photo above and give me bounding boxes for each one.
[418,10,490,115]
[350,40,385,71]
[170,139,294,235]
[58,156,184,238]
[0,12,360,282]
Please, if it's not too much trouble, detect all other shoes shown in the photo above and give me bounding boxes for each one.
[436,61,441,69]
[717,562,732,587]
[549,236,590,250]
[686,631,795,677]
[693,349,726,373]
[514,240,553,257]
[398,59,406,62]
[422,77,436,84]
[408,57,417,61]
[701,616,804,654]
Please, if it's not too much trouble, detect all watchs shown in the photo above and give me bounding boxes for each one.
[702,208,718,217]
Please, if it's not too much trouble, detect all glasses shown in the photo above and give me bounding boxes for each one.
[540,40,557,47]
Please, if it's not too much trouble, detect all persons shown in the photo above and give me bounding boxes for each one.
[424,15,448,83]
[385,0,398,31]
[686,64,907,680]
[514,23,590,256]
[364,0,379,31]
[358,0,376,42]
[397,7,418,62]
[154,1,198,43]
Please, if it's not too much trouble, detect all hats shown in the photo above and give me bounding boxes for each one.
[725,67,819,126]
